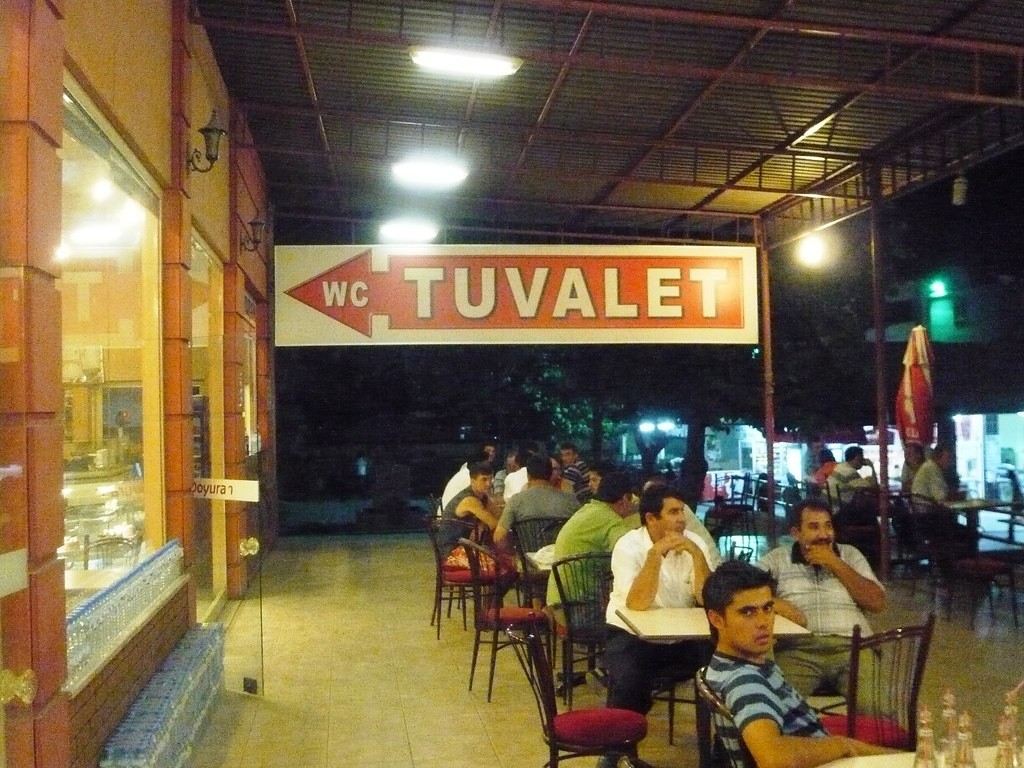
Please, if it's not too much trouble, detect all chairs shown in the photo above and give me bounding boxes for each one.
[418,468,1024,768]
[81,533,145,569]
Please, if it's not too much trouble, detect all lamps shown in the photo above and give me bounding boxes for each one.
[189,107,227,175]
[239,210,265,254]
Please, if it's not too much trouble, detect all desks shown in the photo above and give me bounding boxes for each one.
[613,606,814,767]
[948,499,1019,531]
[525,551,553,573]
[64,568,133,591]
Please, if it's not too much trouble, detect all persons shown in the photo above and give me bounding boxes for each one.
[434,440,734,607]
[701,559,910,768]
[754,496,900,724]
[596,484,717,767]
[546,470,636,695]
[801,432,1024,574]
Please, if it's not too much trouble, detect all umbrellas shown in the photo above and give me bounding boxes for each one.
[895,325,933,450]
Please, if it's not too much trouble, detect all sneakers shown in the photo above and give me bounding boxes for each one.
[585,666,607,704]
[597,752,620,768]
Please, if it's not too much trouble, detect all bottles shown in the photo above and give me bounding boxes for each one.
[914,691,1024,768]
